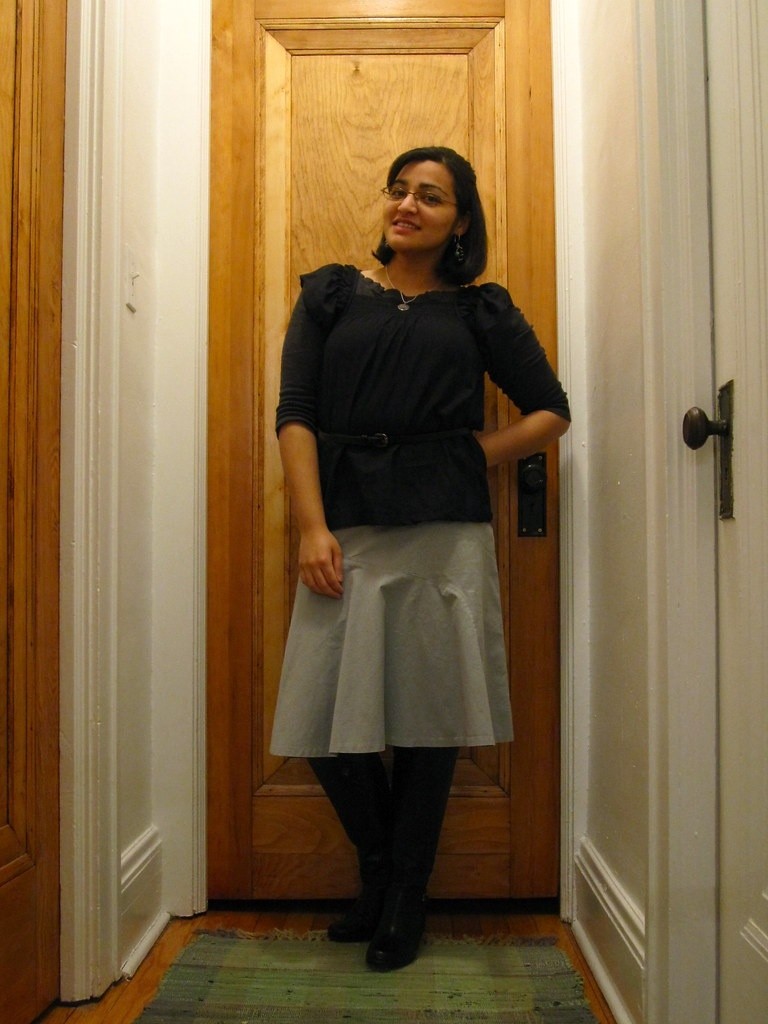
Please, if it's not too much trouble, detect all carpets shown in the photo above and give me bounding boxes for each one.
[132,932,600,1024]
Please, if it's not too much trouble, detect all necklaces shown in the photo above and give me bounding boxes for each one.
[386,265,440,311]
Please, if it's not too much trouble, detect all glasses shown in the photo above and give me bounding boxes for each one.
[381,185,458,207]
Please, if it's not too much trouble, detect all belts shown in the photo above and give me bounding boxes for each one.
[318,428,471,448]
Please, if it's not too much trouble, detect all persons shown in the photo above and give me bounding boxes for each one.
[269,148,571,971]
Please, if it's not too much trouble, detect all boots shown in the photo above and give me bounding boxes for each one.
[312,766,393,943]
[366,759,456,969]
